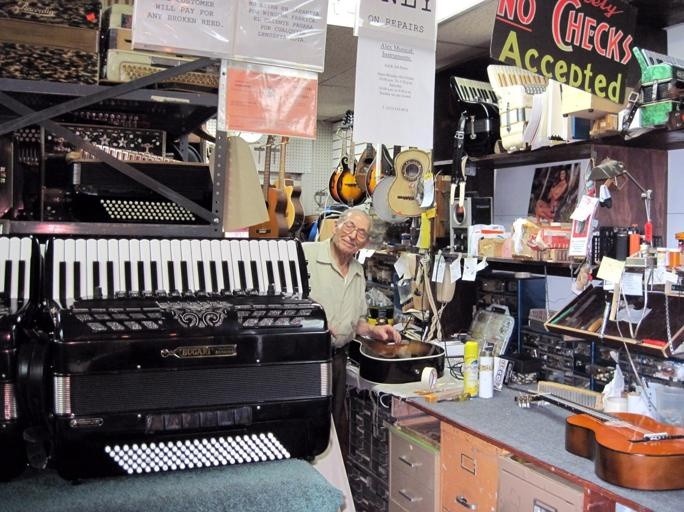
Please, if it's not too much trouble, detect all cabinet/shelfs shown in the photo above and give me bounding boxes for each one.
[342,390,617,512]
[429,0,684,397]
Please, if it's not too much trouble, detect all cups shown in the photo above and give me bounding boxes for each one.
[363,255,394,308]
[616,233,627,261]
[629,231,640,257]
[400,232,410,248]
[479,350,494,398]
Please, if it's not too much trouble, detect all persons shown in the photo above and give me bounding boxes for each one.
[535,169,569,220]
[293,202,403,466]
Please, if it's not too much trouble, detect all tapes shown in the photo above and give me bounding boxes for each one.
[421,365,438,393]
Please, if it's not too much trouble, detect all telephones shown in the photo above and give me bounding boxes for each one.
[436,261,456,304]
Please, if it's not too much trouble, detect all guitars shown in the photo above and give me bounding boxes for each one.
[328,109,432,225]
[347,333,444,385]
[514,391,684,490]
[252,136,304,237]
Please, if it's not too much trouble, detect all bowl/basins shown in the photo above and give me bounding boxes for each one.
[536,248,569,262]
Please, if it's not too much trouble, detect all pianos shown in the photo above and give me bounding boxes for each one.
[447,76,499,105]
[631,46,684,127]
[486,64,550,98]
[0,233,334,485]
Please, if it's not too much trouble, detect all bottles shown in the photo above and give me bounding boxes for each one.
[639,242,649,257]
[669,249,680,268]
[462,340,478,400]
[656,247,666,267]
